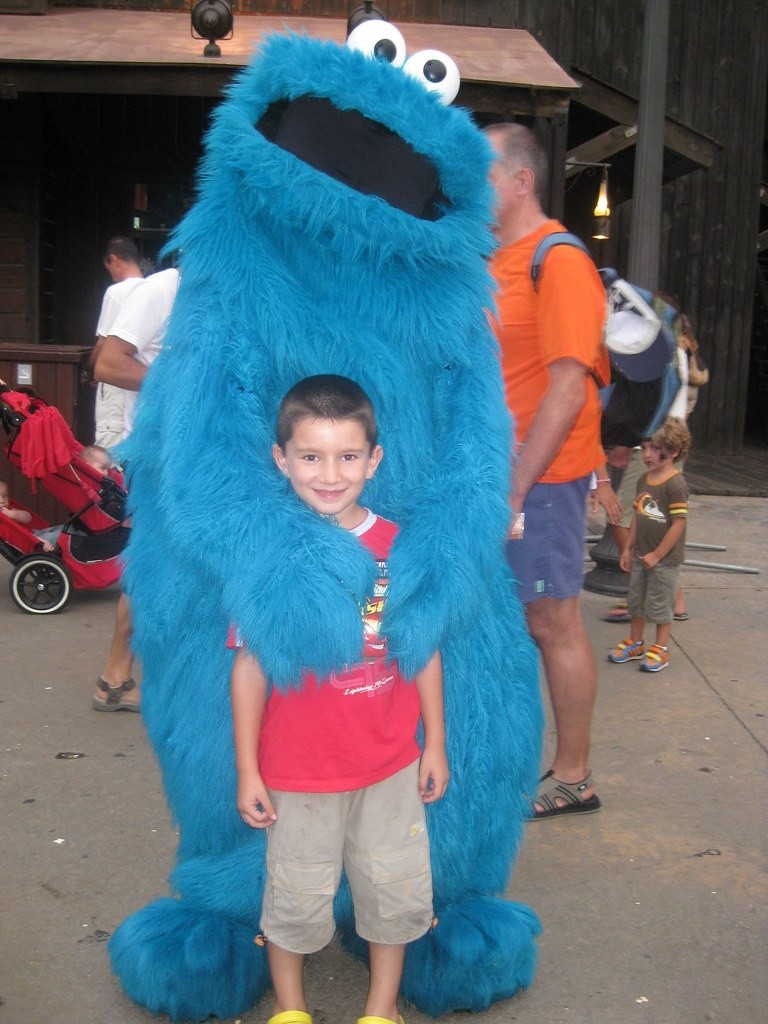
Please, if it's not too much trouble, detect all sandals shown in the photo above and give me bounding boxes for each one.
[525,770,602,821]
[93,675,141,713]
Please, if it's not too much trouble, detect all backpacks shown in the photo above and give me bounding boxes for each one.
[533,231,678,444]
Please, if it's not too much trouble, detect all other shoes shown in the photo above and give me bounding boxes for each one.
[674,593,689,620]
[601,601,632,623]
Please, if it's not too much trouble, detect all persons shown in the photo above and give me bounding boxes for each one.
[93,262,181,438]
[91,235,148,458]
[219,374,454,1024]
[591,282,693,673]
[454,117,608,825]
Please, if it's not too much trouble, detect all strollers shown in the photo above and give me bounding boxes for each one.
[0,381,135,615]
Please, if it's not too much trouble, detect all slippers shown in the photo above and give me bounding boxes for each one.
[265,1010,314,1024]
[356,1012,407,1024]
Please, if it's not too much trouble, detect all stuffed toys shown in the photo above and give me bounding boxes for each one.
[102,14,543,1024]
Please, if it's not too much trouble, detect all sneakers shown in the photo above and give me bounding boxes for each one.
[641,644,671,674]
[606,639,644,664]
